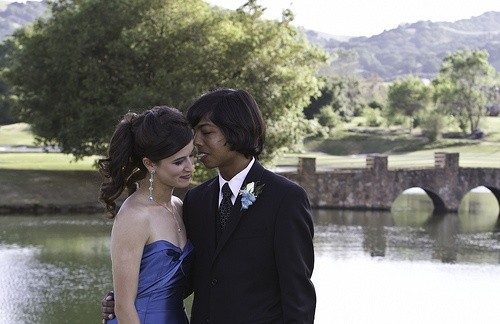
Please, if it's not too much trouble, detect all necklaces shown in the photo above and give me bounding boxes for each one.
[139,187,182,232]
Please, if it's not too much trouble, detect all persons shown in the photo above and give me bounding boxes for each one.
[101,88,316,324]
[98,106,195,324]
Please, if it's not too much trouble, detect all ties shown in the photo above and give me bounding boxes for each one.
[216,183,234,232]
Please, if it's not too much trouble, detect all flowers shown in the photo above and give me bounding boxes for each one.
[239,180,264,211]
